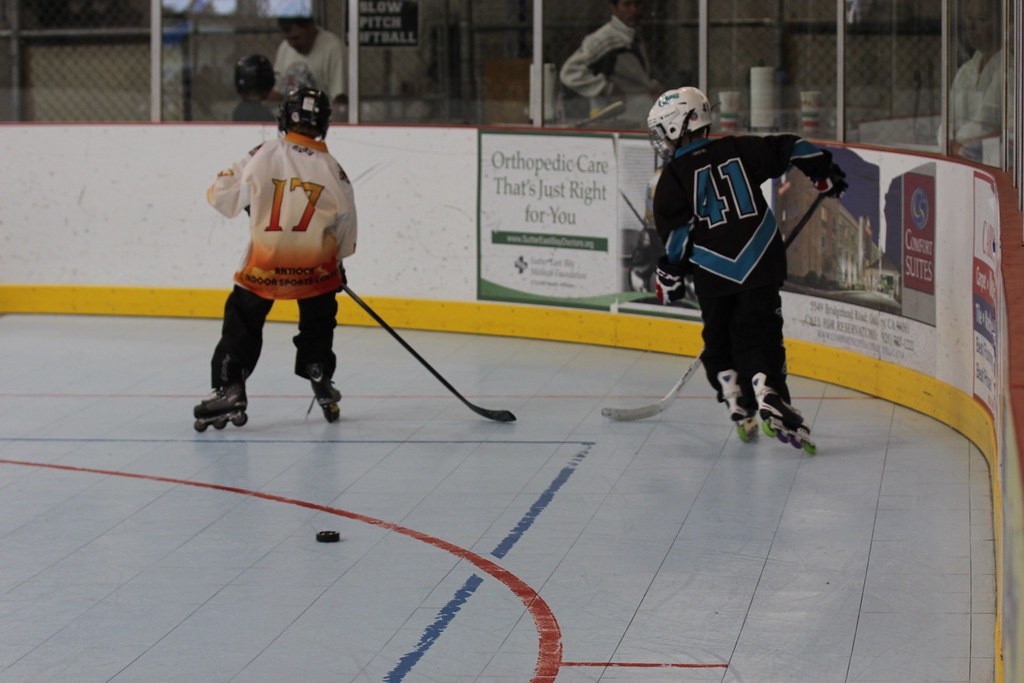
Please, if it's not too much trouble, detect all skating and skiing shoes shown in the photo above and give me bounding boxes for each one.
[752,372,820,456]
[307,354,344,422]
[715,368,758,443]
[194,355,249,431]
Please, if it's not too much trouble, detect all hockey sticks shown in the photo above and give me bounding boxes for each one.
[341,281,518,422]
[601,193,826,422]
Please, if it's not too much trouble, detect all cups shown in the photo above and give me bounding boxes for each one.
[798,90,822,138]
[718,90,740,133]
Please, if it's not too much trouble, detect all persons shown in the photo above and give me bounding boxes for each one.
[194,87,357,433]
[232,54,282,122]
[938,0,1003,156]
[559,0,659,122]
[648,86,849,454]
[262,17,347,121]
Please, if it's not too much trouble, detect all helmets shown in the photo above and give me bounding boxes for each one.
[646,86,712,160]
[234,52,276,101]
[279,87,329,141]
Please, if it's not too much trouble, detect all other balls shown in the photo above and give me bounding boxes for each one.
[317,531,340,543]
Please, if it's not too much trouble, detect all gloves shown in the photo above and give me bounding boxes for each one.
[809,164,848,198]
[654,268,686,304]
